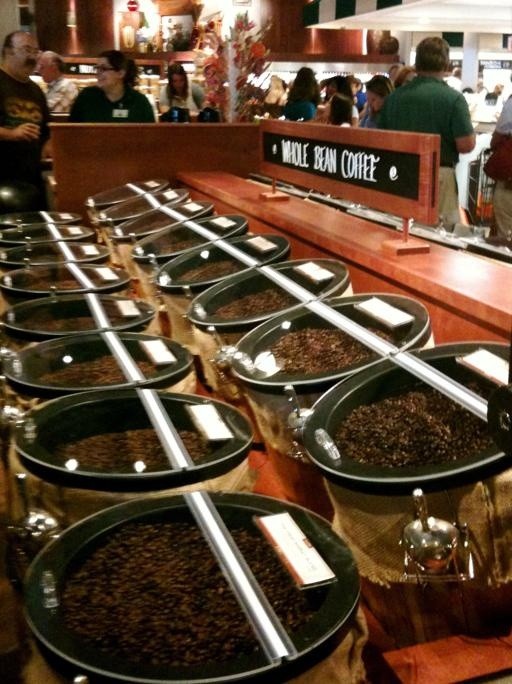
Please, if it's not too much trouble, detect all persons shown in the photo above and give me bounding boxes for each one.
[68,49,155,122]
[1,28,53,213]
[261,35,511,249]
[158,63,211,116]
[36,50,81,113]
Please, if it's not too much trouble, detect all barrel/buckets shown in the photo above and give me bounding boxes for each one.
[161,14,194,52]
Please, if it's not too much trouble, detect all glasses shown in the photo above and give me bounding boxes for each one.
[93,64,117,72]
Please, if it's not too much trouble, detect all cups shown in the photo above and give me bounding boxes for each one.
[137,74,170,114]
